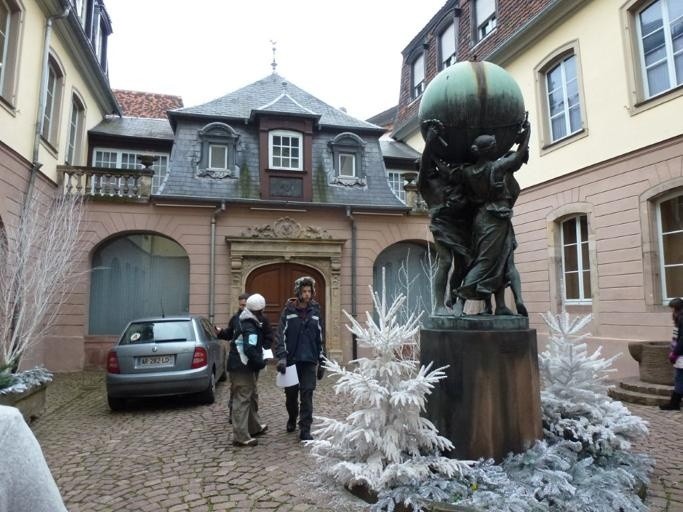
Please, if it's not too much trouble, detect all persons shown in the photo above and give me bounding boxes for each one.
[227,293,267,447]
[274,276,329,441]
[213,294,275,424]
[413,117,528,315]
[660,297,683,410]
[0,403,67,512]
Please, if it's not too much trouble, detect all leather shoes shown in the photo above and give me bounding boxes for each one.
[300,430,313,440]
[287,416,296,432]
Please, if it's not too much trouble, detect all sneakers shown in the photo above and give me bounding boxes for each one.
[233,437,258,446]
[250,424,268,436]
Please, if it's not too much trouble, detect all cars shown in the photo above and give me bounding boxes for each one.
[104,295,231,411]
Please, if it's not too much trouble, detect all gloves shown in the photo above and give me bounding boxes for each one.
[276,353,287,374]
[317,362,325,379]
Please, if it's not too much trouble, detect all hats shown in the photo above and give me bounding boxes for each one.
[294,276,316,301]
[246,293,266,312]
